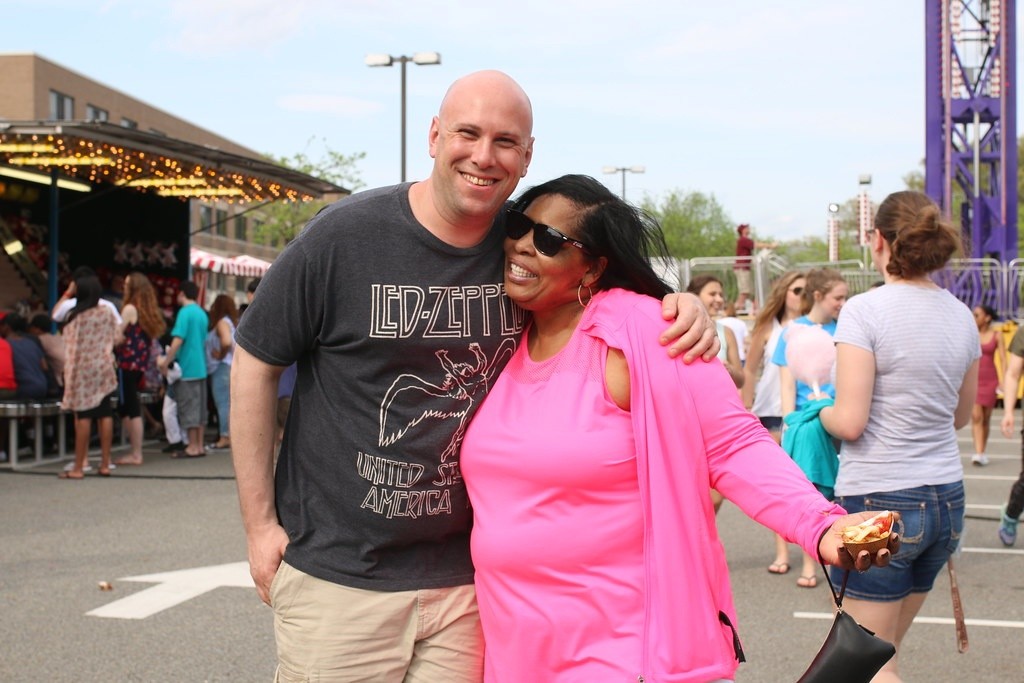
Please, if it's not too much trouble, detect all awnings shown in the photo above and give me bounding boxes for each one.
[1,121,354,205]
[188,249,273,276]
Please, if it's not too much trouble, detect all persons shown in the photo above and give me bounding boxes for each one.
[0,272,247,479]
[230,70,720,683]
[206,294,238,449]
[733,224,780,309]
[248,277,298,463]
[808,190,982,683]
[454,173,900,683]
[744,271,808,575]
[972,304,1009,466]
[771,268,849,586]
[718,304,751,368]
[112,274,167,465]
[0,338,18,463]
[156,280,206,458]
[684,273,744,519]
[1001,325,1024,547]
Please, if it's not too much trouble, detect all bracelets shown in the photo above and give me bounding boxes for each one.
[64,291,72,298]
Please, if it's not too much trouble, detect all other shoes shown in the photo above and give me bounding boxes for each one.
[113,457,143,466]
[205,444,231,452]
[972,454,988,466]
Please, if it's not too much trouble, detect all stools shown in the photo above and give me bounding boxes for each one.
[0,393,160,461]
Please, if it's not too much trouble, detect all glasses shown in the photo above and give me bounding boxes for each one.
[505,207,600,256]
[788,286,802,294]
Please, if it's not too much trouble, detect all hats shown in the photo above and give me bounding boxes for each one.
[737,225,748,234]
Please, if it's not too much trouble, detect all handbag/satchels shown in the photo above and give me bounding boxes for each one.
[796,526,896,683]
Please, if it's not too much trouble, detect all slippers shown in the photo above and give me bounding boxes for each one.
[58,471,84,479]
[97,468,111,476]
[199,452,206,456]
[172,449,201,457]
[768,563,790,574]
[797,573,816,587]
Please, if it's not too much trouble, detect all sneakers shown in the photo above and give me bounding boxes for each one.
[999,504,1018,545]
